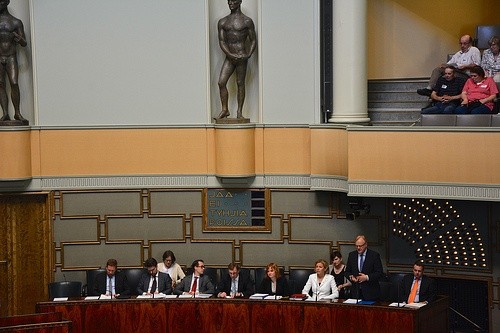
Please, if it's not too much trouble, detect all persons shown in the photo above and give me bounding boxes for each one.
[399,262,432,304]
[213,0,257,119]
[0,0,28,122]
[344,234,383,301]
[301,259,339,299]
[328,251,352,299]
[417,34,500,115]
[92,242,277,297]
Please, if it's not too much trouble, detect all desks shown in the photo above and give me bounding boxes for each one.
[9,298,451,333]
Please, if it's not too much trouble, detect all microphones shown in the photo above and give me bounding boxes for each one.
[62,272,71,301]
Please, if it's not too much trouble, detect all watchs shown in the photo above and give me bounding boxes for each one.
[239,292,242,297]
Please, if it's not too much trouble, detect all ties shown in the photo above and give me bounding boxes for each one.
[360,253,365,272]
[232,279,236,291]
[150,276,157,294]
[109,277,112,295]
[191,276,199,292]
[407,278,418,304]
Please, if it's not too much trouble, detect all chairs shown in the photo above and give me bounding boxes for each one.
[474,25,500,49]
[220,269,250,280]
[254,266,284,293]
[47,282,81,299]
[186,268,217,283]
[290,269,315,293]
[81,270,105,296]
[121,268,144,294]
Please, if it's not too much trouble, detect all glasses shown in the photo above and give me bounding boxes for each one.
[198,265,205,269]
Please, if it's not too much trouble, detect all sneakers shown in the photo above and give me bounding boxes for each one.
[417,88,433,97]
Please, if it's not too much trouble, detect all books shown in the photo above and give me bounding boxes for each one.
[249,293,282,300]
[137,294,177,299]
[84,294,115,300]
[178,294,213,298]
[389,302,426,308]
[52,297,69,301]
[343,299,374,305]
[288,294,331,302]
